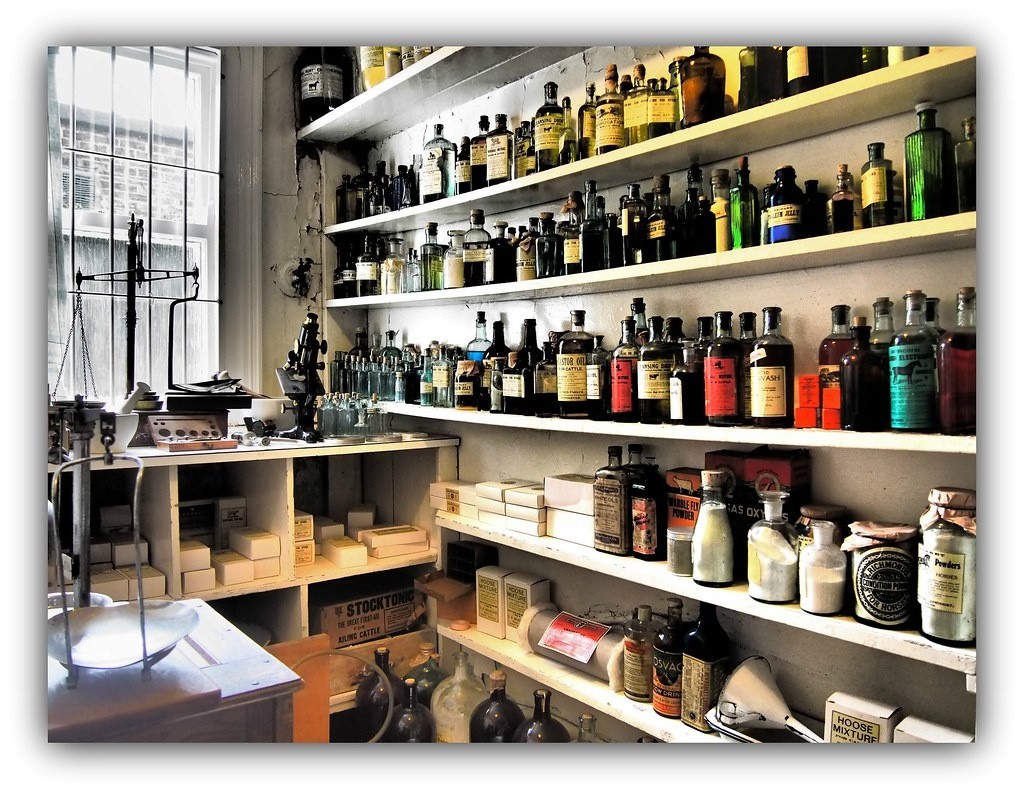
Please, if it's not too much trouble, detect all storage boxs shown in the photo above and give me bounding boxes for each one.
[294,502,428,566]
[824,690,899,745]
[546,509,595,546]
[476,567,513,640]
[429,478,547,537]
[314,585,428,646]
[544,465,597,516]
[893,714,975,744]
[667,444,808,577]
[504,571,551,643]
[74,504,165,601]
[179,495,280,593]
[316,624,440,697]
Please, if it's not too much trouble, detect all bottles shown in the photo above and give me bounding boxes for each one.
[511,689,571,743]
[593,443,666,561]
[568,712,612,743]
[386,678,435,743]
[799,520,848,615]
[624,604,663,703]
[469,669,525,743]
[666,525,695,576]
[293,44,976,434]
[354,647,406,743]
[430,650,490,743]
[851,485,976,648]
[399,642,450,709]
[680,601,730,734]
[748,489,799,605]
[652,597,685,719]
[793,503,847,553]
[692,468,734,588]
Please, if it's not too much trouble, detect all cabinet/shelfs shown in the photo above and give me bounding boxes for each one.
[46,430,458,712]
[297,47,976,454]
[434,516,977,744]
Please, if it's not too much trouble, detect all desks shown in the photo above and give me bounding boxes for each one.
[48,602,303,739]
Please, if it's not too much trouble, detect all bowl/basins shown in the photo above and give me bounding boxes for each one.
[251,398,296,432]
